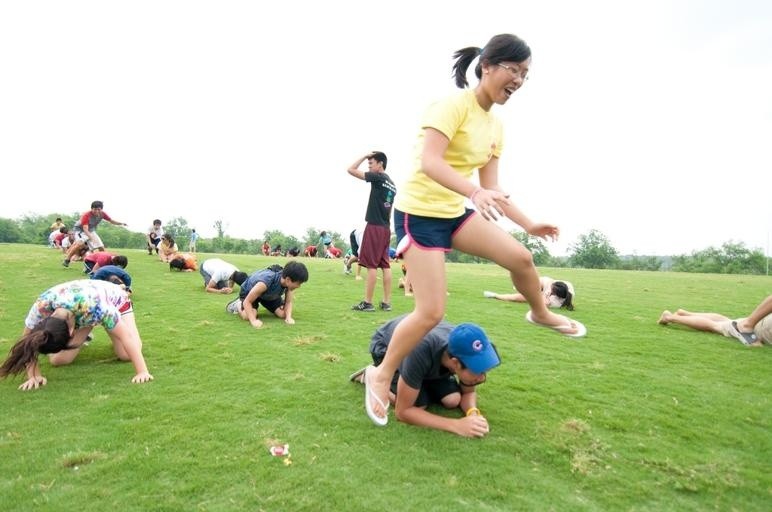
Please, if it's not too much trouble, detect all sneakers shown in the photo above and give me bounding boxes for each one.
[381,302,391,311]
[63,259,70,267]
[484,291,495,298]
[352,301,375,311]
[227,296,240,312]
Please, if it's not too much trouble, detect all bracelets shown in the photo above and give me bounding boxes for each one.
[466,407,481,417]
[470,185,484,203]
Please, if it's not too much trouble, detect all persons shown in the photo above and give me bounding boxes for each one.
[146,221,414,328]
[48,201,132,289]
[365,33,588,427]
[348,149,399,311]
[350,310,501,439]
[728,294,772,348]
[658,308,772,343]
[0,278,154,391]
[484,276,576,309]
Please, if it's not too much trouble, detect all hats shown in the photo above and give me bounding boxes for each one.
[447,324,499,373]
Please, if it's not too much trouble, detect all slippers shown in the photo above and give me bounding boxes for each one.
[349,369,364,384]
[526,310,586,337]
[730,321,763,347]
[366,366,390,426]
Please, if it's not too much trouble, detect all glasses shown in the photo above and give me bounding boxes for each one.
[496,62,528,81]
